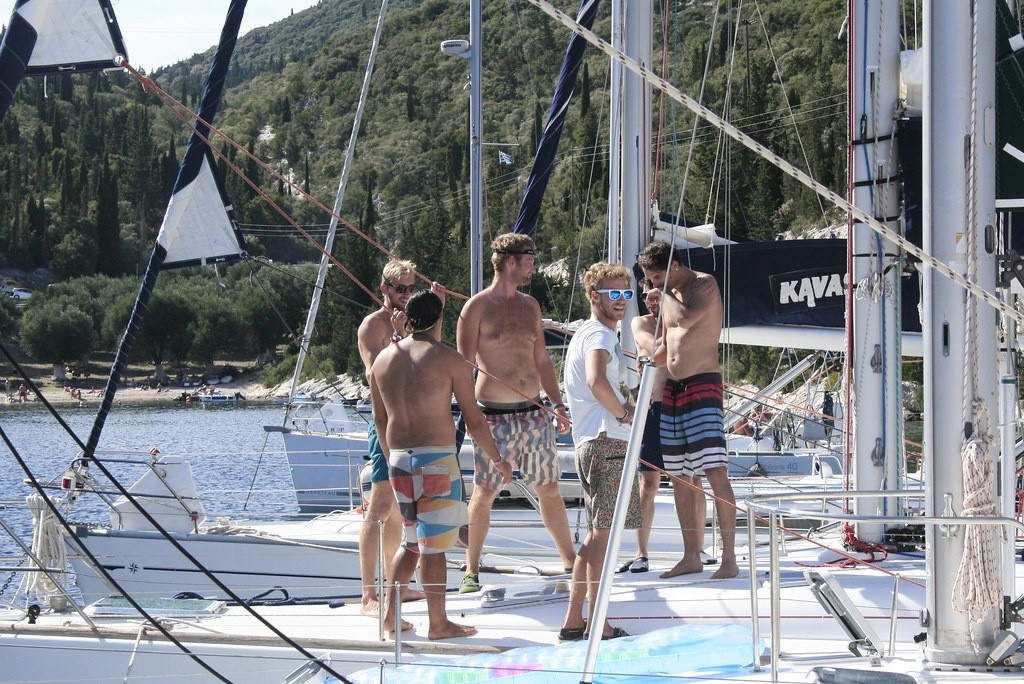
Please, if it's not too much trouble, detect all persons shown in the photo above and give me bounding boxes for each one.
[190,381,219,395]
[63,385,111,400]
[371,290,514,641]
[630,240,739,579]
[455,233,579,593]
[559,261,631,643]
[356,258,445,608]
[729,417,754,437]
[4,376,46,403]
[126,374,170,392]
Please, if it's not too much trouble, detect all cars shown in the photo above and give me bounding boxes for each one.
[9,288,34,299]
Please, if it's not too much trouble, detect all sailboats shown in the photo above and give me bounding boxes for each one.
[0,0,1024,684]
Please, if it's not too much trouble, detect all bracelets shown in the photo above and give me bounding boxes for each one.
[390,332,402,342]
[616,409,630,428]
[491,454,503,465]
[553,403,565,409]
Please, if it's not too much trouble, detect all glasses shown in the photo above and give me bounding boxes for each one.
[596,289,634,301]
[640,293,647,302]
[384,281,417,294]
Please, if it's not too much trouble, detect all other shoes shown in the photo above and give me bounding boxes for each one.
[629,556,649,573]
[699,550,717,565]
[559,621,586,640]
[459,573,480,593]
[583,627,630,640]
[565,567,573,579]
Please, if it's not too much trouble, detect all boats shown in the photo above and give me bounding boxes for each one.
[197,394,236,402]
